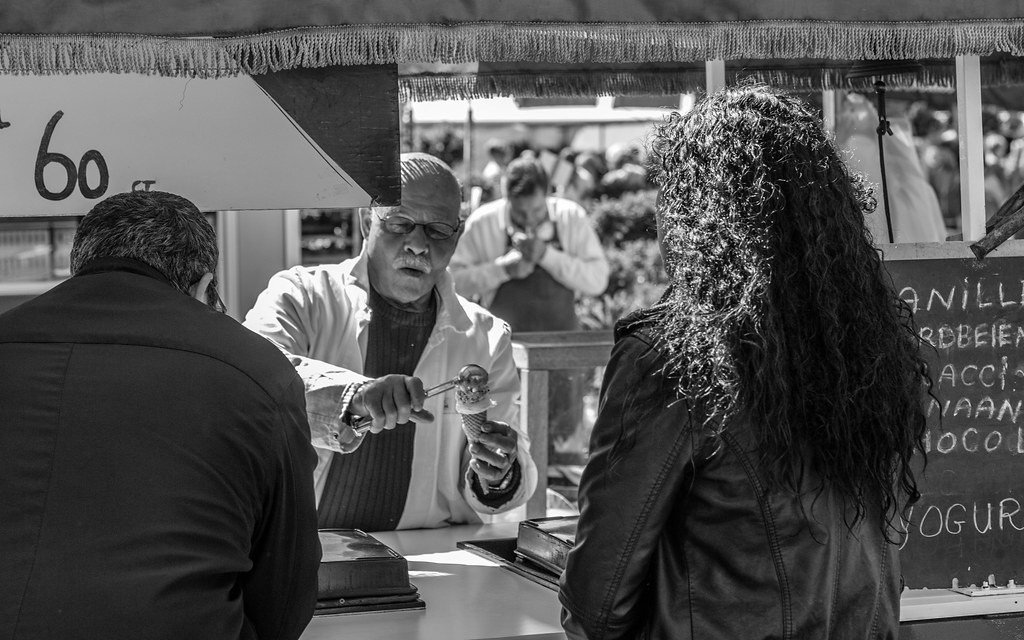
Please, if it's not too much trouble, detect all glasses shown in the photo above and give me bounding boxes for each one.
[372,207,461,240]
[216,297,227,314]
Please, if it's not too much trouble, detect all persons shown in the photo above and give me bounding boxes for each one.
[451,159,609,448]
[833,94,1024,243]
[397,134,670,326]
[0,192,322,640]
[241,152,538,531]
[558,81,943,640]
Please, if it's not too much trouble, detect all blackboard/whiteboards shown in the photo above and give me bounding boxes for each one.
[858,237,1024,623]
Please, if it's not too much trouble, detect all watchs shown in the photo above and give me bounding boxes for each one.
[488,470,513,490]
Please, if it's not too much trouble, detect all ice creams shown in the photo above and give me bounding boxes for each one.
[455,378,497,494]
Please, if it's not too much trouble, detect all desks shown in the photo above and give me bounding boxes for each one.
[299,523,1024,640]
